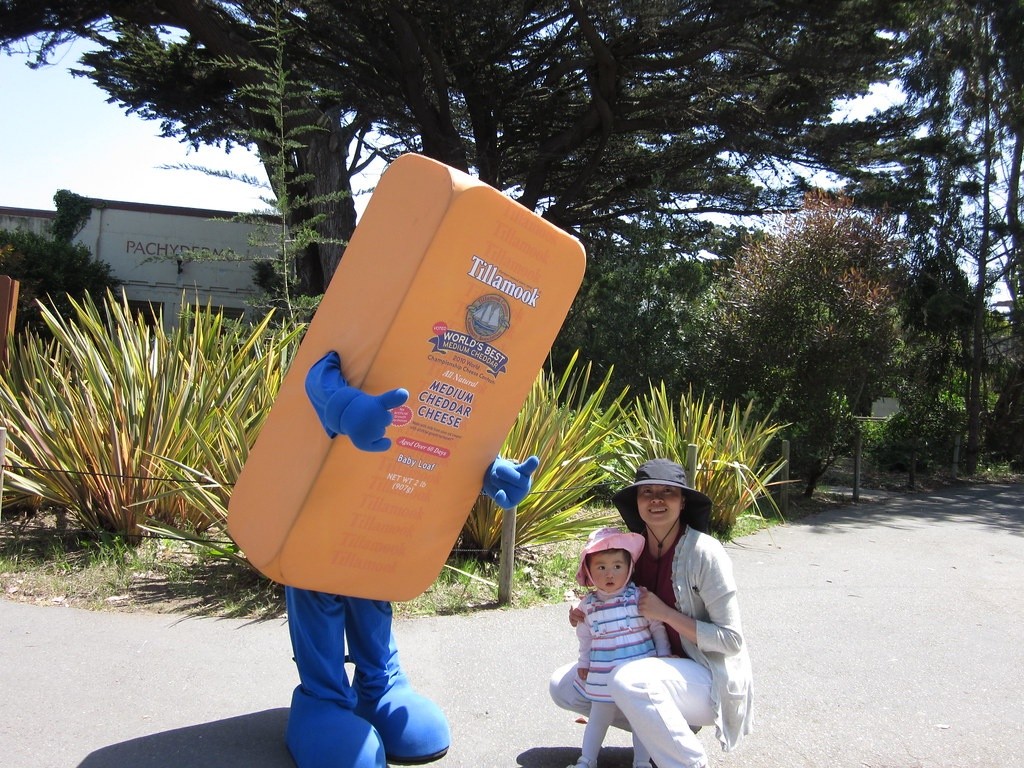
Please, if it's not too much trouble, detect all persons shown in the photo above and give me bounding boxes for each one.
[549,458,753,768]
[565,528,681,768]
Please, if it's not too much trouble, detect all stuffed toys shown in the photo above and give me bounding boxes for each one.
[226,152,588,768]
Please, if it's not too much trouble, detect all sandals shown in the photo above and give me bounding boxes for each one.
[633,762,652,768]
[566,756,597,768]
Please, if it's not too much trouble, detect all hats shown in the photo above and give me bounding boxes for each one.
[612,459,712,533]
[575,527,645,587]
